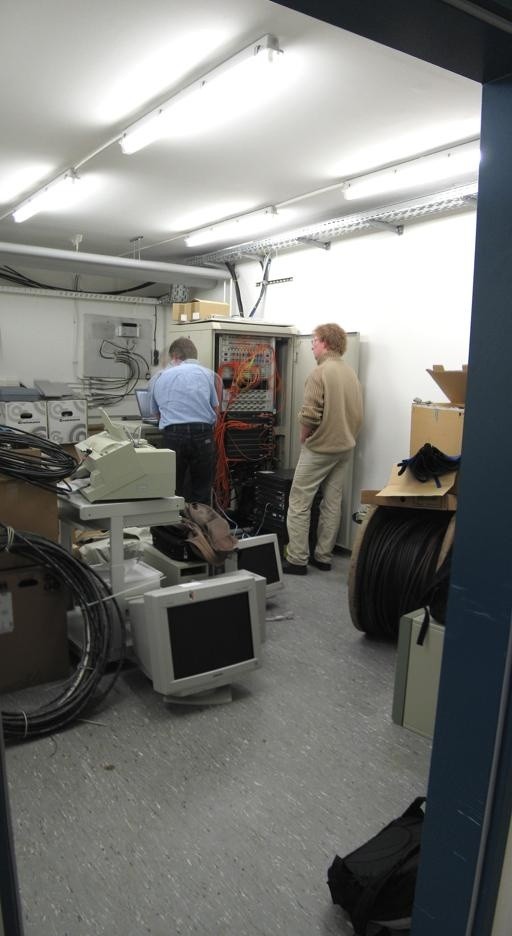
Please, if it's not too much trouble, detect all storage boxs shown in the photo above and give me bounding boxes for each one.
[0,476,65,569]
[0,398,49,440]
[45,396,89,446]
[172,301,192,322]
[0,562,75,695]
[189,296,231,322]
[375,361,470,501]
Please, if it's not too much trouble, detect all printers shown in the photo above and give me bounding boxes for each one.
[72,405,178,504]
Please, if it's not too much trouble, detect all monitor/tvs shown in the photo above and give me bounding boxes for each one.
[126,576,265,708]
[226,532,288,602]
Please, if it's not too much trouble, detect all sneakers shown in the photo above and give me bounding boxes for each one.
[308,556,331,571]
[283,563,308,575]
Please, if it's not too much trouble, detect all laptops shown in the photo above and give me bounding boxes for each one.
[134,388,159,425]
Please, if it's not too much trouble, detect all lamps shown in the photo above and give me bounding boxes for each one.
[338,137,483,203]
[10,165,77,227]
[184,201,284,249]
[113,30,287,160]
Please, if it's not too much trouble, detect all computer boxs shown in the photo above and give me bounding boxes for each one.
[192,568,268,643]
[391,605,446,739]
[139,542,209,586]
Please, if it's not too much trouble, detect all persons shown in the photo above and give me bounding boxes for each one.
[147,337,220,506]
[281,324,364,575]
